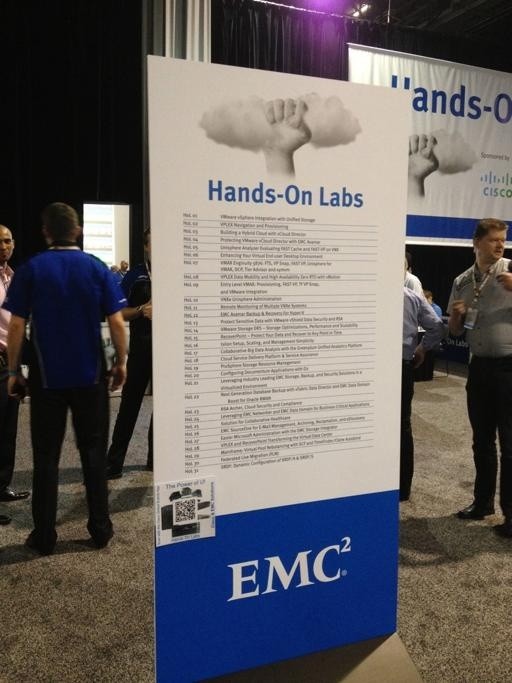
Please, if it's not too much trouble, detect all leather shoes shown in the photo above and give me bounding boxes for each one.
[460,503,496,519]
[25,535,55,556]
[0,489,29,501]
[103,462,122,480]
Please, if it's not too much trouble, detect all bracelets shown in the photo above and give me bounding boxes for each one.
[8,369,19,376]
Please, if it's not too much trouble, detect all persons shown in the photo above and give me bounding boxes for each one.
[101,229,152,481]
[1,223,31,526]
[110,264,119,275]
[446,217,510,538]
[399,284,445,500]
[1,199,129,552]
[255,94,310,181]
[421,288,443,378]
[404,252,427,303]
[408,131,443,197]
[117,259,129,280]
